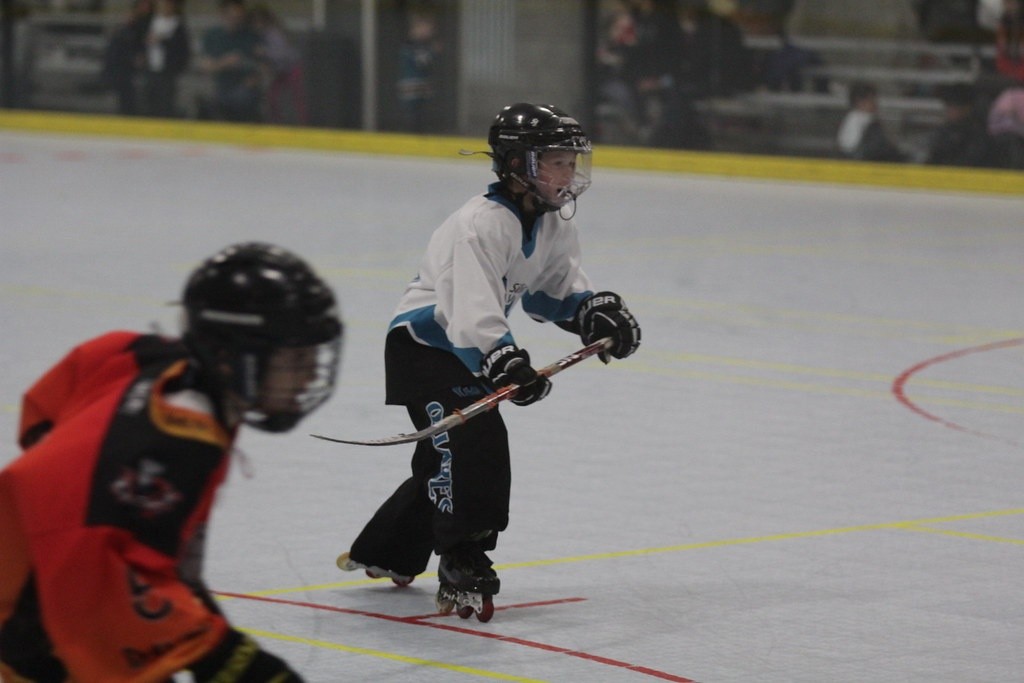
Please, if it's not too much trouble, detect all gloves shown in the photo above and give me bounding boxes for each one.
[480,345,552,407]
[192,628,303,683]
[577,291,642,365]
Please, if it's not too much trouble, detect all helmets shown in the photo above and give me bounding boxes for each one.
[182,242,342,433]
[487,102,593,209]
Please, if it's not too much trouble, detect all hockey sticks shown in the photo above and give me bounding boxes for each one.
[309,336,615,449]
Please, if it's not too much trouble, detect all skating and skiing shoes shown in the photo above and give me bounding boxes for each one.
[337,533,428,586]
[437,547,499,623]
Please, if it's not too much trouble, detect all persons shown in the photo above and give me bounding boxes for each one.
[597,0,827,155]
[836,77,910,163]
[1,244,350,683]
[89,0,191,119]
[916,0,1024,169]
[390,0,450,132]
[174,0,310,124]
[337,102,641,623]
